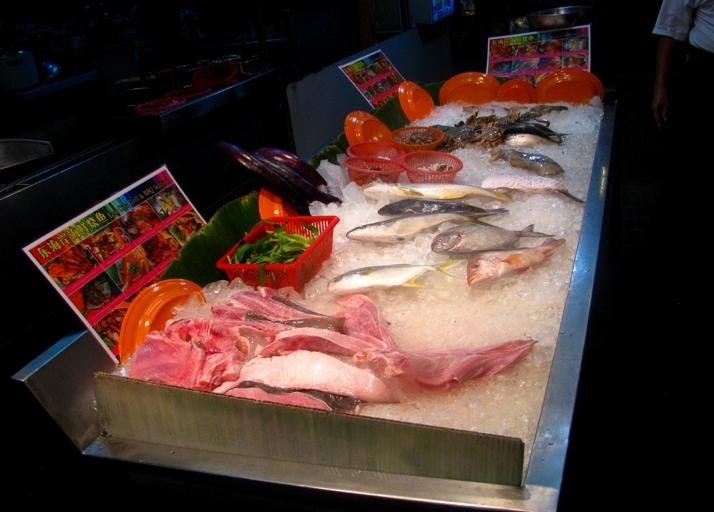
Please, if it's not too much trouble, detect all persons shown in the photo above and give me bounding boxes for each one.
[651,0,713,131]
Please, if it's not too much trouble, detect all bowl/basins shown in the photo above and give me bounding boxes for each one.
[526,7,588,29]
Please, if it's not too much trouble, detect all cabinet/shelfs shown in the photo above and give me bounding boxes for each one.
[9,68,622,512]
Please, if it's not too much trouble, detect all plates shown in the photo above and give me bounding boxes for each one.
[397,79,435,122]
[535,69,604,105]
[437,72,501,107]
[118,278,204,366]
[258,186,300,219]
[497,79,535,104]
[343,108,391,149]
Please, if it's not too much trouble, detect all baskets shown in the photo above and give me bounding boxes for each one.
[217,215,340,292]
[344,157,405,185]
[389,126,445,153]
[346,142,405,163]
[397,150,463,184]
